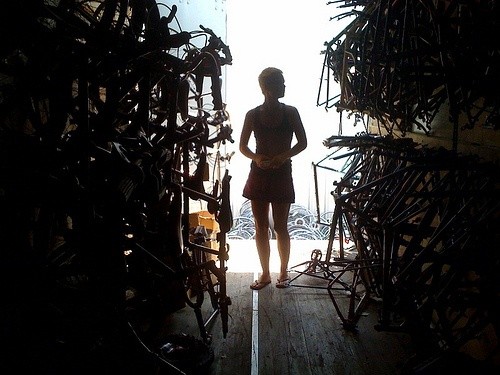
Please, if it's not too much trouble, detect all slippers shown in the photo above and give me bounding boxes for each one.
[275,276,291,288]
[248,279,272,290]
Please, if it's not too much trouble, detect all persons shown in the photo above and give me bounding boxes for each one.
[239,68,308,290]
